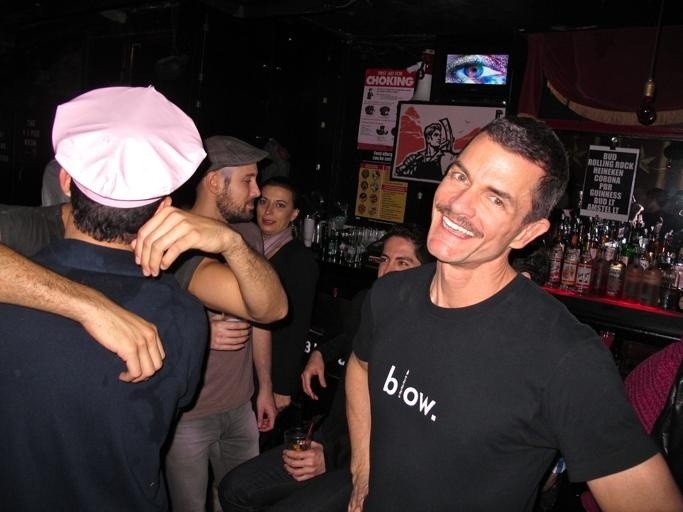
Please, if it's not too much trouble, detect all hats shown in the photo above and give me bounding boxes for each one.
[203,134,270,175]
[50,85,209,211]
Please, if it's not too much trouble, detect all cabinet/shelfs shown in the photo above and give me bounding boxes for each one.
[309,262,378,336]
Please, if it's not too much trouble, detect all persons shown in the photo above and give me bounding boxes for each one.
[640,187,670,234]
[395,116,462,181]
[215,222,427,512]
[0,79,327,511]
[337,110,683,512]
[570,335,681,512]
[446,55,509,85]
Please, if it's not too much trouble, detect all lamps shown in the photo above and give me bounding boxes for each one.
[636,0,665,125]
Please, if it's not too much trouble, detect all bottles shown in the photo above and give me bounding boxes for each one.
[302,215,381,265]
[547,214,683,311]
[413,47,436,102]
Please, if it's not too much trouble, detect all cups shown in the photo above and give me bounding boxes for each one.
[283,426,311,451]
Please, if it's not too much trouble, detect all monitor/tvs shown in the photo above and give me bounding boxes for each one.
[429,40,511,106]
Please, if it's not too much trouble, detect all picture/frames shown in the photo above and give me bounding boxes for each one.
[388,101,510,185]
